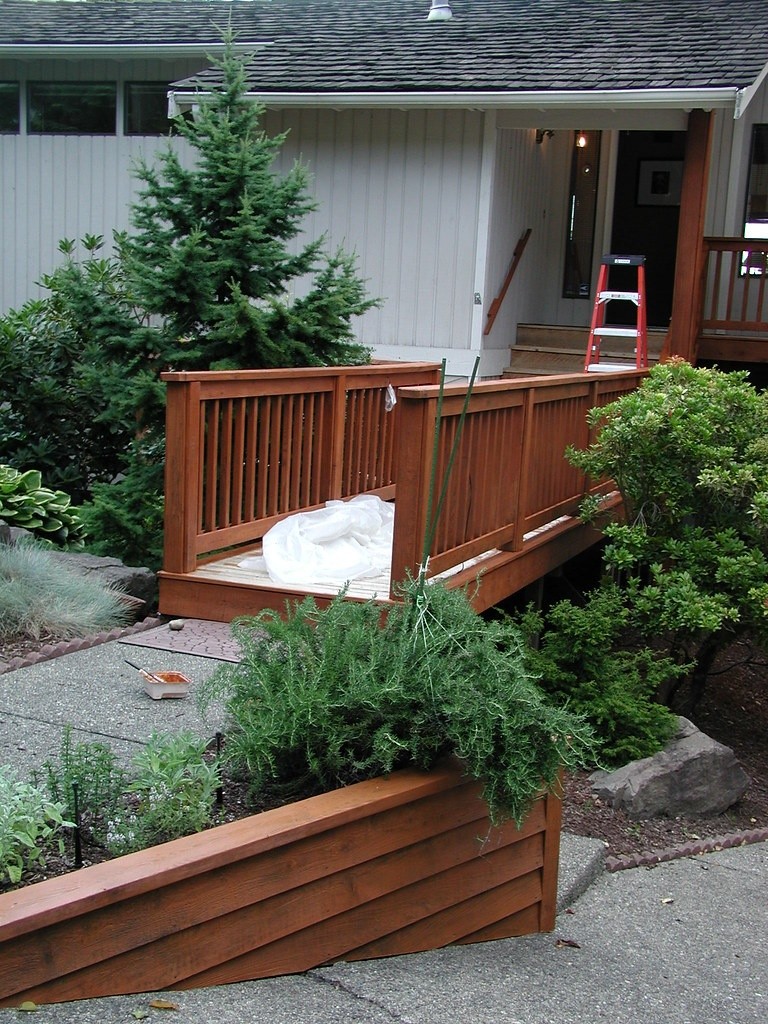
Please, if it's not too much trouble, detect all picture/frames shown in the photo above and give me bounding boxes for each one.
[634,158,685,208]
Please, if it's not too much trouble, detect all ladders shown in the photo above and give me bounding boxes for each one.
[584,255,648,375]
